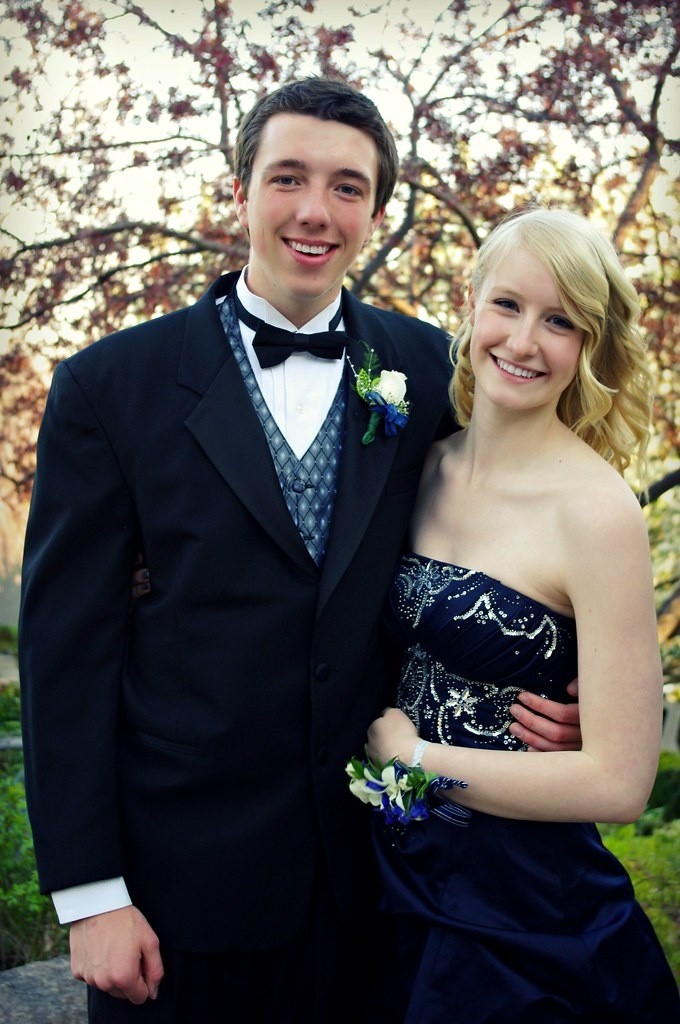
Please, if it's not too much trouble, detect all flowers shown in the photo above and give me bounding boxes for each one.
[353,339,409,446]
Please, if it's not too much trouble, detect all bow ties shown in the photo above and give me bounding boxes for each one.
[234,288,348,369]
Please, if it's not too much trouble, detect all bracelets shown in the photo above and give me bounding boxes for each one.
[412,740,428,767]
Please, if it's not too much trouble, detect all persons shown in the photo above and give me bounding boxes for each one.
[343,211,680,1024]
[18,76,582,1024]
